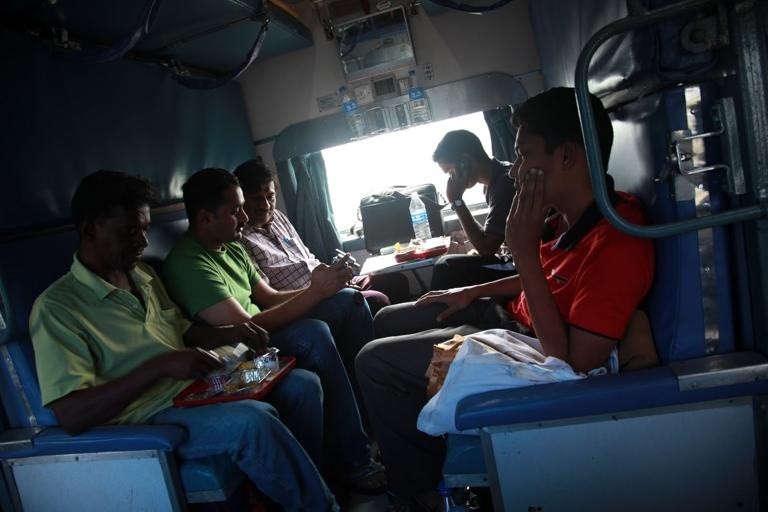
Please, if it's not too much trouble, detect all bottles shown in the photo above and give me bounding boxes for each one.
[437,481,467,512]
[408,191,432,244]
[407,69,432,125]
[338,85,367,140]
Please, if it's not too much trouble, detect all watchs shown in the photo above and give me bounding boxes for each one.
[451,199,464,211]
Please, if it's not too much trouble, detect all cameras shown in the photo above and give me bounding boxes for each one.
[331,248,360,276]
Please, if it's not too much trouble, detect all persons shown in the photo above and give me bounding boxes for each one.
[28,168,342,511]
[159,167,396,494]
[431,129,528,291]
[233,156,373,352]
[353,86,659,512]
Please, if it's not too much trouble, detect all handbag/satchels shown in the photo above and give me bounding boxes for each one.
[416,311,658,441]
[360,184,445,255]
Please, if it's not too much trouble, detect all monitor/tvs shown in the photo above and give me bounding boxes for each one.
[331,5,417,83]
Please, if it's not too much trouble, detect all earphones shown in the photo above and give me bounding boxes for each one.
[561,155,569,165]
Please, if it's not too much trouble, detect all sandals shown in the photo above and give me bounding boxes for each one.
[391,490,442,512]
[350,455,390,493]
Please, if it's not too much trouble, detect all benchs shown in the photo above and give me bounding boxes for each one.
[0,206,241,511]
[443,0,768,511]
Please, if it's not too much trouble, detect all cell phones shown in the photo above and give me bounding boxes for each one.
[458,157,469,176]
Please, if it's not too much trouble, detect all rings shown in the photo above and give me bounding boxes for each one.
[341,258,345,264]
